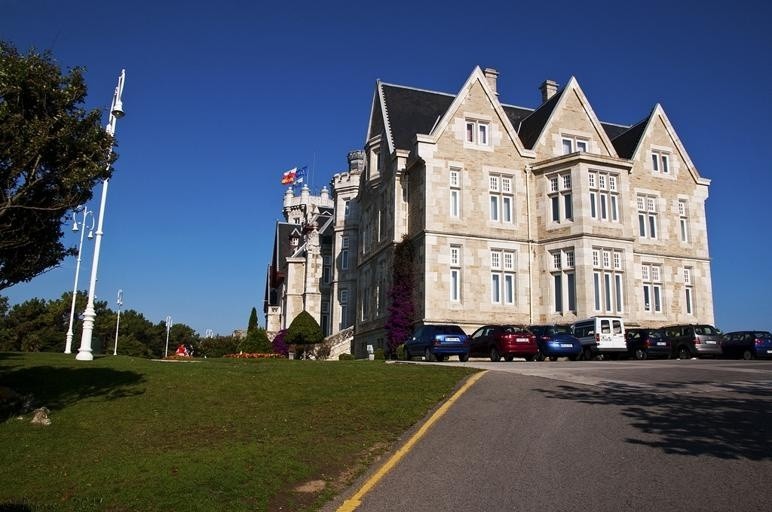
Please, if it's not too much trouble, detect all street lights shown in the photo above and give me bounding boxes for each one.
[164,315,173,358]
[64,67,128,362]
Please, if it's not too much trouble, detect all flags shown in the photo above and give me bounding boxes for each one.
[281,167,304,190]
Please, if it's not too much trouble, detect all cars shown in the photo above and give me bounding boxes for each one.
[467,325,540,362]
[526,324,584,362]
[570,316,772,361]
[403,325,470,362]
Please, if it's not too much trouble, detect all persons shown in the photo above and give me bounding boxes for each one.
[175,344,194,356]
